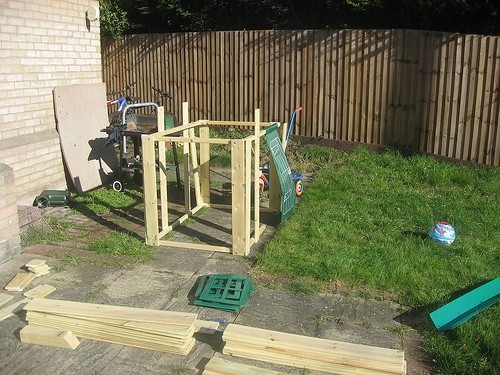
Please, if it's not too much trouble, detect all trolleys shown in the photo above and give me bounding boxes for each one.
[111,101,184,193]
[252,106,304,197]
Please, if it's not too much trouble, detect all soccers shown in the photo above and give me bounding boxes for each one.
[428,221,456,247]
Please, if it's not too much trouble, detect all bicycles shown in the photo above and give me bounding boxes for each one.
[149,86,177,131]
[107,82,139,128]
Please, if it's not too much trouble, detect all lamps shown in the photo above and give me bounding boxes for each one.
[85,6,102,23]
